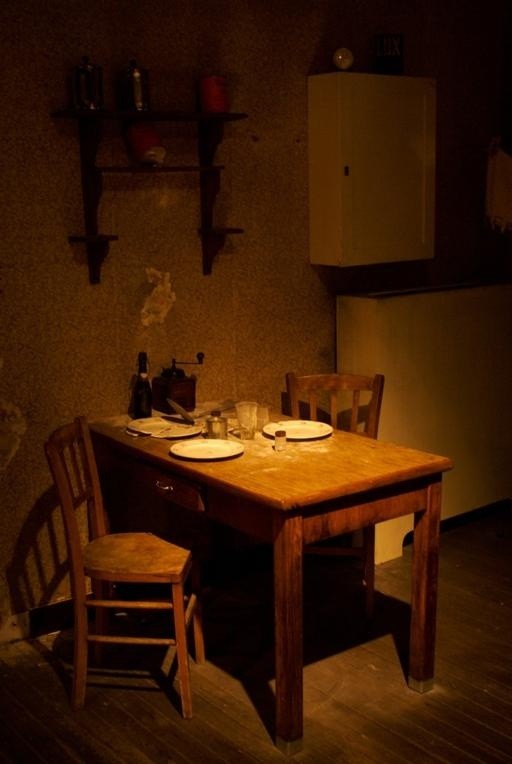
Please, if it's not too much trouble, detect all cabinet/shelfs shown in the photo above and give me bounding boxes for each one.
[305,71,438,267]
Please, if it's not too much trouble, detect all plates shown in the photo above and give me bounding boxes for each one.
[262,418,334,442]
[167,438,247,461]
[124,416,203,439]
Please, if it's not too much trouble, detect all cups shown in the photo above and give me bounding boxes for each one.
[234,401,258,442]
[198,71,232,114]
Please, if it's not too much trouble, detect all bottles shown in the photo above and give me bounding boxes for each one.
[121,59,152,114]
[151,352,204,414]
[274,430,287,455]
[128,351,155,418]
[204,410,229,441]
[70,53,105,112]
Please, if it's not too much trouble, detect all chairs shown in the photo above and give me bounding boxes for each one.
[259,370,385,621]
[44,414,206,721]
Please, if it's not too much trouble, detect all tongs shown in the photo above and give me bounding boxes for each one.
[162,398,195,425]
